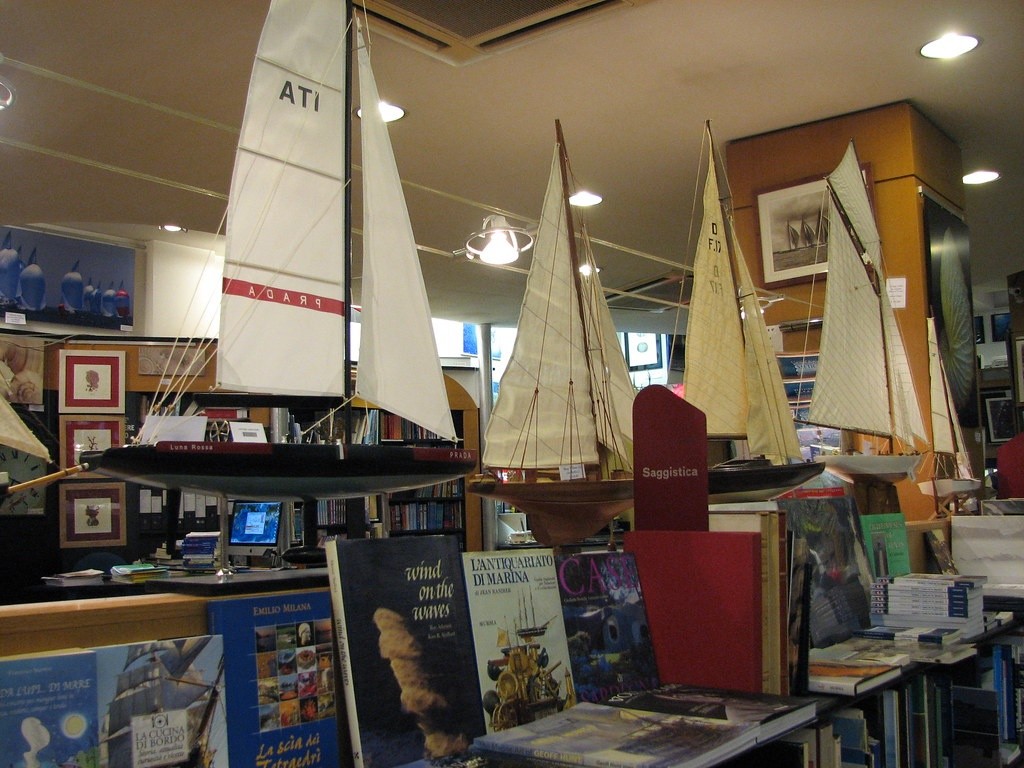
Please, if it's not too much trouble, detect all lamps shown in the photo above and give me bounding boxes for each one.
[465,213,536,265]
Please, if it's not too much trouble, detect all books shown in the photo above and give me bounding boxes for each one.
[0,409,1024,768]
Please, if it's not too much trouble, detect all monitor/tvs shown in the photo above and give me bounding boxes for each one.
[228,501,282,573]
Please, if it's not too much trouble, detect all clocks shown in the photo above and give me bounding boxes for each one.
[0,444,46,515]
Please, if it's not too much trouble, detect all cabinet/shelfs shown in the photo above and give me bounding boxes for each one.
[303,406,469,550]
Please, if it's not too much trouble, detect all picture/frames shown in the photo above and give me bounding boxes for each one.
[974,316,985,344]
[984,397,1015,443]
[60,482,127,548]
[60,415,126,479]
[623,331,663,373]
[991,312,1012,342]
[58,351,126,414]
[751,161,878,291]
